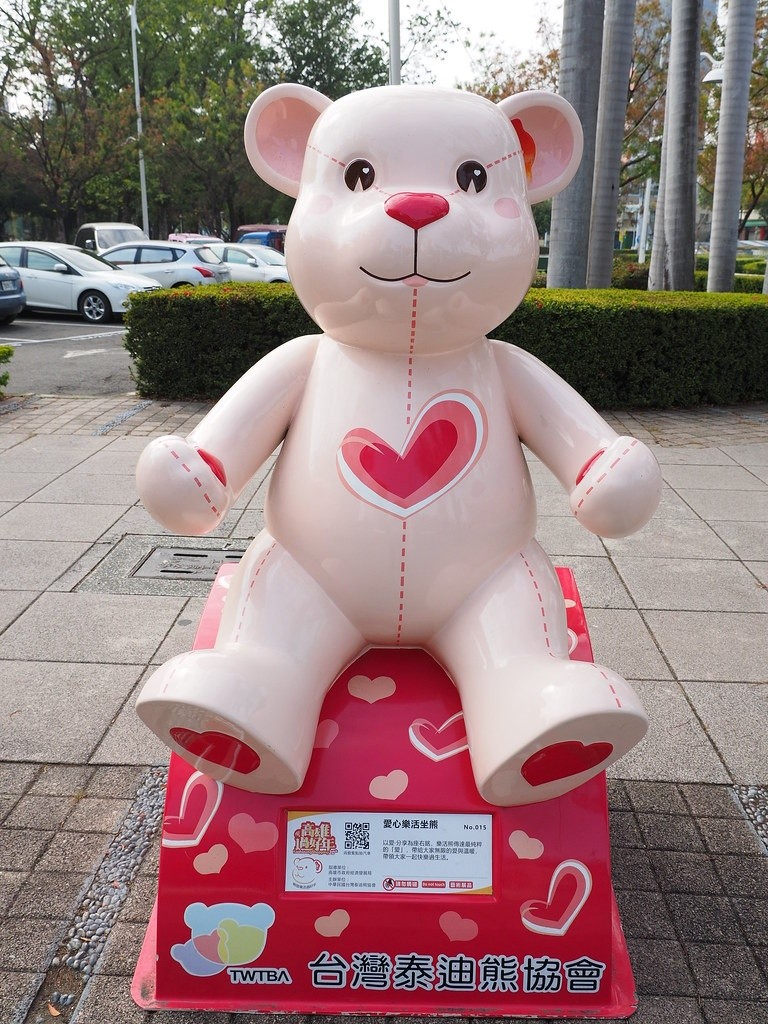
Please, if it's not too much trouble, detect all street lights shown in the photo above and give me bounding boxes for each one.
[636,49,724,265]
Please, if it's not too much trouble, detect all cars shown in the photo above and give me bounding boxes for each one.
[0,240,164,325]
[203,242,290,285]
[0,252,27,331]
[236,230,286,254]
[183,237,225,245]
[96,240,232,290]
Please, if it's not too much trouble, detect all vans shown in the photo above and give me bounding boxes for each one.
[73,222,150,256]
[168,230,203,242]
